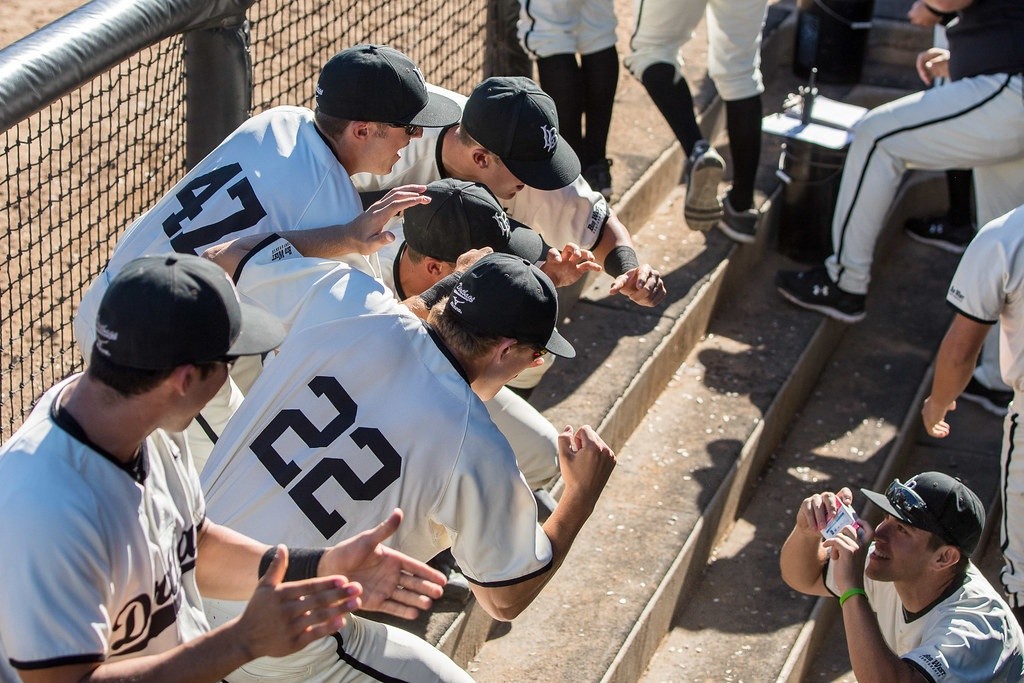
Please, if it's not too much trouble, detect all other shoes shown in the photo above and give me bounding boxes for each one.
[585,158,620,200]
[717,185,776,246]
[682,140,734,231]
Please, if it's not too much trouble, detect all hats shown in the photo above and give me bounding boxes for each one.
[443,252,577,357]
[94,252,288,360]
[315,44,463,128]
[398,177,547,268]
[859,472,988,561]
[461,76,583,190]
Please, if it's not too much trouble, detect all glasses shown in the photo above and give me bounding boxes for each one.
[886,475,938,523]
[391,122,419,135]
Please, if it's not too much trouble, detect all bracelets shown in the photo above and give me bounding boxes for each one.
[260,541,324,585]
[603,246,638,275]
[838,588,869,606]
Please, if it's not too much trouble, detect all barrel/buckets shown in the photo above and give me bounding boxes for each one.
[773,137,850,263]
[791,0,877,86]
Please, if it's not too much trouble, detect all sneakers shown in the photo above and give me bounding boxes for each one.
[958,373,1017,419]
[772,258,867,324]
[903,213,984,255]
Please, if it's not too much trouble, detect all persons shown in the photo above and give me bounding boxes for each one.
[200,183,616,683]
[0,254,448,683]
[921,203,1024,630]
[774,0,1024,419]
[512,0,620,205]
[622,0,769,244]
[73,44,666,493]
[776,471,1024,683]
